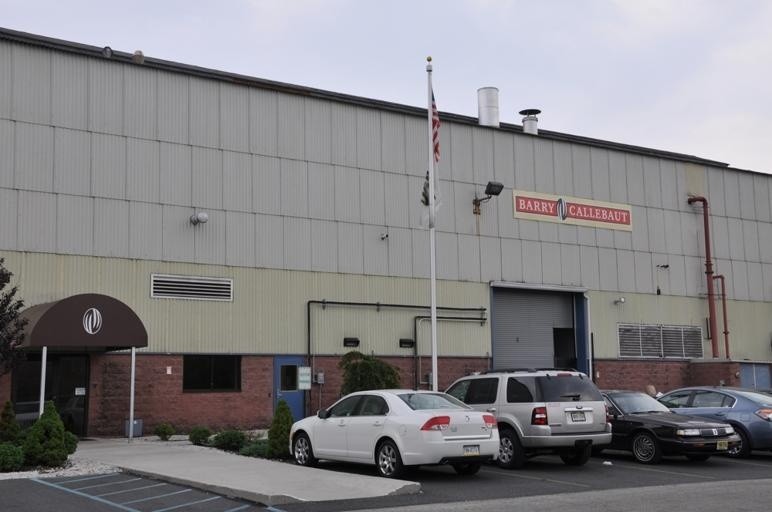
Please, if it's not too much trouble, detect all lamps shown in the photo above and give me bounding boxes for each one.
[190,212,208,225]
[473,181,504,206]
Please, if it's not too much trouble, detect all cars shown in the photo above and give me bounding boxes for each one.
[288,387,503,478]
[14,394,86,436]
[599,388,741,465]
[655,385,771,459]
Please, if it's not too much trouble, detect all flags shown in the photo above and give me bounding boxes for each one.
[420,90,441,209]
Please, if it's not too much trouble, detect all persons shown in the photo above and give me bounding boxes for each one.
[646,385,664,398]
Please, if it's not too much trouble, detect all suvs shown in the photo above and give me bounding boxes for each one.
[442,366,615,471]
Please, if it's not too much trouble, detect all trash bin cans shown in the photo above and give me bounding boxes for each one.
[125,418,144,437]
